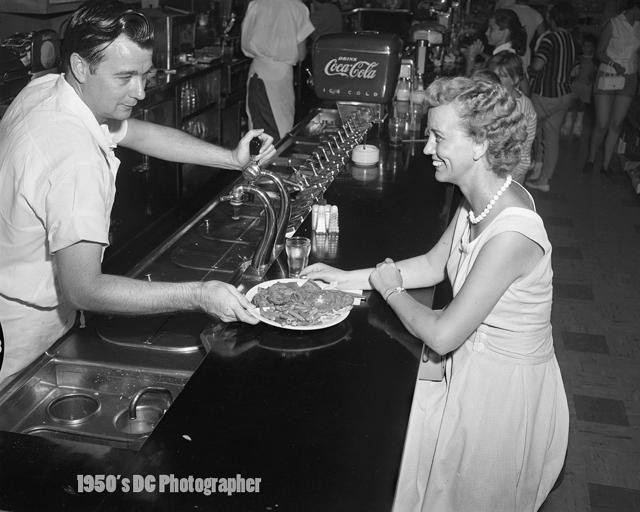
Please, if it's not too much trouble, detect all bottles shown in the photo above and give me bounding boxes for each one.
[310,197,340,235]
[395,101,410,121]
[397,77,409,102]
[310,233,339,261]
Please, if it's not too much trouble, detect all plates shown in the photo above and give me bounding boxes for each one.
[257,317,350,352]
[243,278,353,329]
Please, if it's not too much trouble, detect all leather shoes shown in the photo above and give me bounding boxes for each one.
[525,182,550,192]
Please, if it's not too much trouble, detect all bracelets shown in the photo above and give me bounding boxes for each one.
[384,286,407,303]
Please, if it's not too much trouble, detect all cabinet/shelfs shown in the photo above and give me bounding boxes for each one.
[177,67,227,190]
[120,96,181,219]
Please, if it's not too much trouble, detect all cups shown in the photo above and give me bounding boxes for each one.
[410,90,423,113]
[387,149,405,172]
[387,114,407,149]
[408,114,421,133]
[285,238,312,276]
[182,117,207,140]
[180,79,201,105]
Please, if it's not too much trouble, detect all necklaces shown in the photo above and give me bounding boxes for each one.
[468,172,513,226]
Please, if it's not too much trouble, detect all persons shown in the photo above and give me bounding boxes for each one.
[0,1,278,393]
[494,1,640,194]
[240,2,317,145]
[494,53,538,185]
[463,8,526,91]
[309,1,344,41]
[296,74,570,512]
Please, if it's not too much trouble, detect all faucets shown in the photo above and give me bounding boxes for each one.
[0,105,378,450]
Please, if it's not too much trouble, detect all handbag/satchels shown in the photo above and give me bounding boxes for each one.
[598,76,626,91]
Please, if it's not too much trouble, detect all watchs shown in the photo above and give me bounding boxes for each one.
[467,56,476,62]
